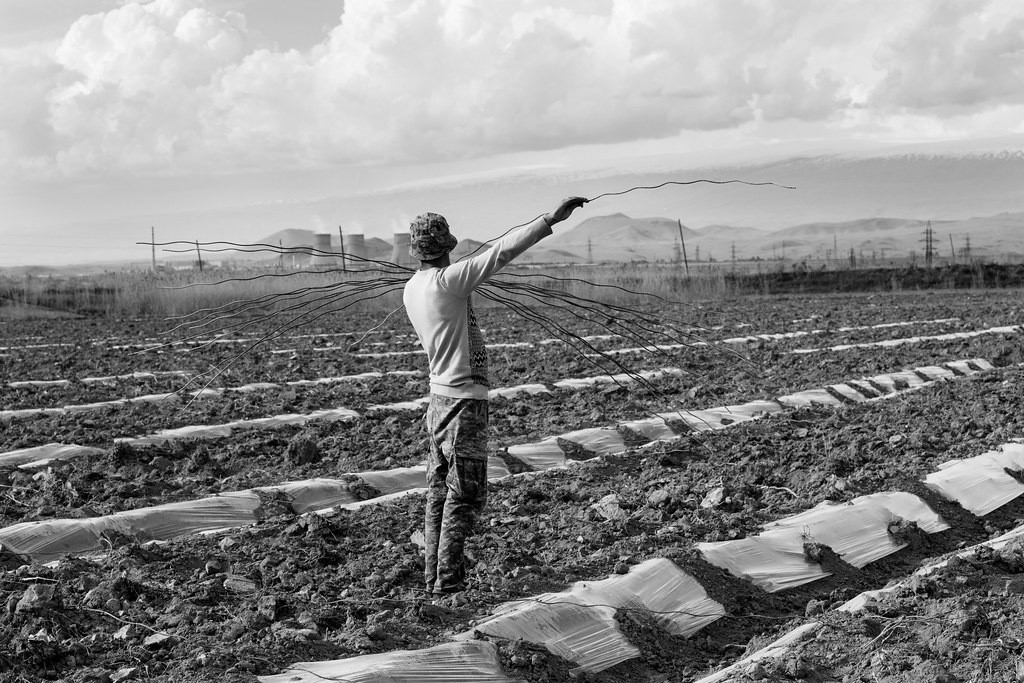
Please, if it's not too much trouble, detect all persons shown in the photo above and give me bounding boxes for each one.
[403,196,590,596]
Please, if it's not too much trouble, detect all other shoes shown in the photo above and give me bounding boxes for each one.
[426,586,467,599]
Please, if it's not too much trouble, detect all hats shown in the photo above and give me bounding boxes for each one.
[409,213,457,260]
